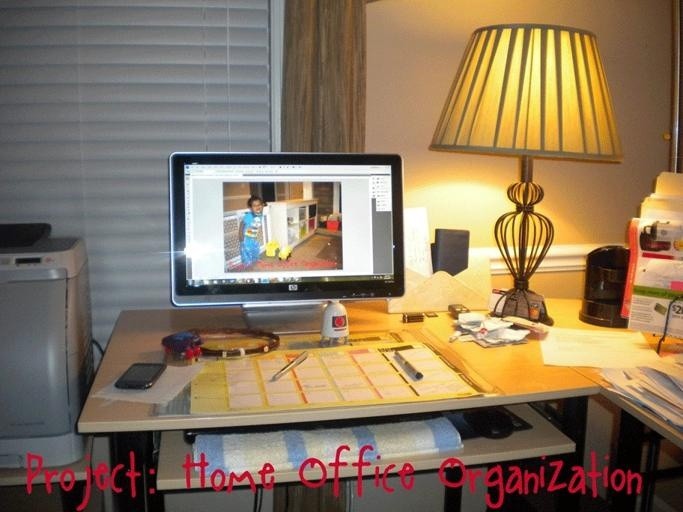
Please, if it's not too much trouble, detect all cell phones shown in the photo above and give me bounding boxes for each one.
[114,363,167,389]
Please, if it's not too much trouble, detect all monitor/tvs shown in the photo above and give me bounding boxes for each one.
[168,151,404,334]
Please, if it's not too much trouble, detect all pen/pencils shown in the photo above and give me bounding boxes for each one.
[272,350,309,381]
[395,351,423,380]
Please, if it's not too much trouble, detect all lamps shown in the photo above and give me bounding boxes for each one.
[429,24,622,335]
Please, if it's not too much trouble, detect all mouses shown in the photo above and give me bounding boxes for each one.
[463,406,514,439]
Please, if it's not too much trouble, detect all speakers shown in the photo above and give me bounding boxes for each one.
[432,227,470,280]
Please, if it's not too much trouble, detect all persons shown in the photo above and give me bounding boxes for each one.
[236,195,263,270]
[159,332,202,369]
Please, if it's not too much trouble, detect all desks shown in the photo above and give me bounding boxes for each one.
[76,311,683,508]
